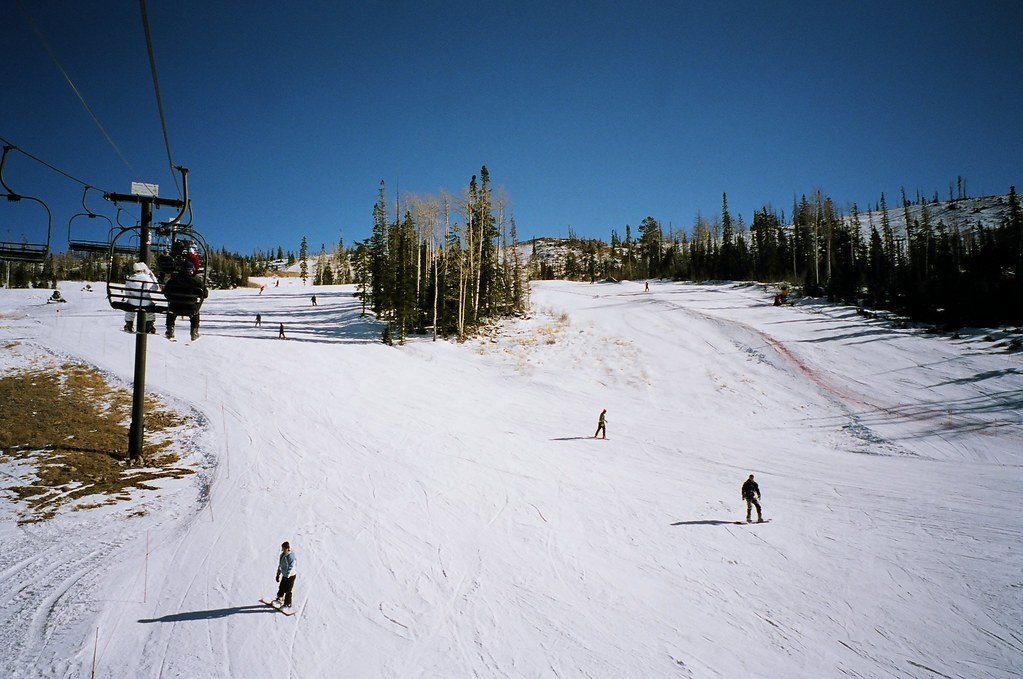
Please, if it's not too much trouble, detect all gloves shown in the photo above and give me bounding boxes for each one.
[276,575,280,583]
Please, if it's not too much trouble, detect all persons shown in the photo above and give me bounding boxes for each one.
[311,294,318,306]
[279,322,286,340]
[645,282,649,292]
[595,409,608,439]
[255,313,261,328]
[773,293,782,306]
[742,475,763,523]
[275,279,279,287]
[259,286,264,294]
[124,239,208,341]
[272,542,296,611]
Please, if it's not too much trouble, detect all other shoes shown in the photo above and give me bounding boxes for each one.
[747,519,752,523]
[273,597,292,609]
[758,519,763,522]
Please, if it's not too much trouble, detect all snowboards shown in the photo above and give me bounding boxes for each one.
[588,436,610,440]
[736,520,770,525]
[262,596,297,615]
